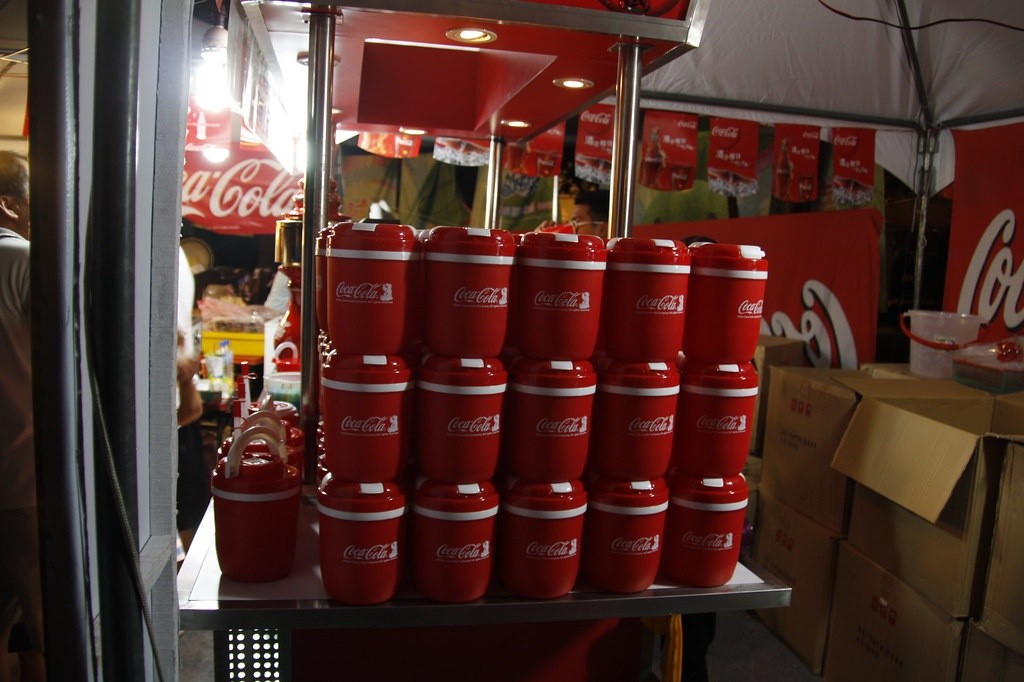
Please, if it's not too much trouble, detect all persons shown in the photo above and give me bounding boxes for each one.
[574,189,610,238]
[0,151,206,682]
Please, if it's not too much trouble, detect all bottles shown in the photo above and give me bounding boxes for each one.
[219,339,235,396]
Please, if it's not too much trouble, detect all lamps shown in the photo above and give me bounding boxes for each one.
[200,7,228,59]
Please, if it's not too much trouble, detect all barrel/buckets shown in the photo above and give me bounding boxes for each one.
[901,311,983,378]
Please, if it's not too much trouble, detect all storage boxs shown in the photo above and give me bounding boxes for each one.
[743,335,1024,682]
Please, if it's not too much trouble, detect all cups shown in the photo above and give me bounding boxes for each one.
[209,218,767,605]
